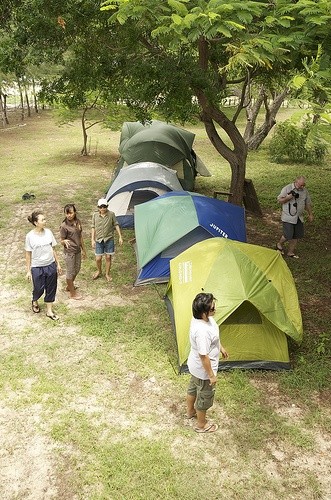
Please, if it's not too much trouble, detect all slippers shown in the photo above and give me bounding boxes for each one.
[287,252,299,258]
[46,312,59,320]
[32,300,40,313]
[276,243,285,255]
[69,292,84,300]
[66,284,78,291]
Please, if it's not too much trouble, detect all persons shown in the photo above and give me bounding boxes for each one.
[276,176,314,259]
[25,210,62,321]
[91,198,123,283]
[183,292,230,433]
[59,204,87,300]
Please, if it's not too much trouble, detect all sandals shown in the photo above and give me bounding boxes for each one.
[184,408,197,419]
[193,423,218,433]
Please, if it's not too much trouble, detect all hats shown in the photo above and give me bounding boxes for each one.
[97,199,108,207]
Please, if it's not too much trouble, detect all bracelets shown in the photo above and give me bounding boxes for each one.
[209,374,214,378]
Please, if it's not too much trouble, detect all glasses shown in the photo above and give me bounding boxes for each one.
[209,303,215,312]
[100,205,107,208]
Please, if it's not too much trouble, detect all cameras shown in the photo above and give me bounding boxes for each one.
[288,190,299,199]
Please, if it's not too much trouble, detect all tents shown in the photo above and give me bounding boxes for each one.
[160,237,304,373]
[129,191,248,286]
[114,119,211,192]
[104,161,184,229]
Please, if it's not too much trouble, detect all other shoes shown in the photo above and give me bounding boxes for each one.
[105,274,113,281]
[93,271,102,280]
